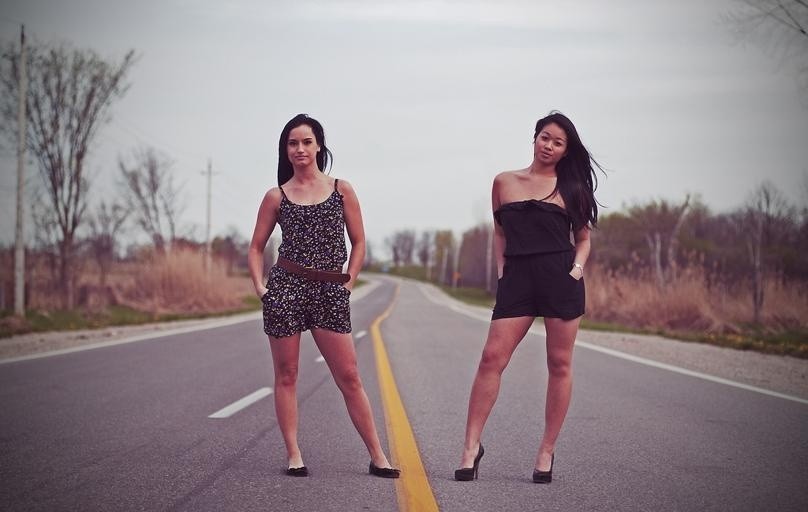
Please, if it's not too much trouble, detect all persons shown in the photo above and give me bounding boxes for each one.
[455,110,607,484]
[247,114,402,478]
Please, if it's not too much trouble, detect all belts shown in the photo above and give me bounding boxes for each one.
[276,255,350,283]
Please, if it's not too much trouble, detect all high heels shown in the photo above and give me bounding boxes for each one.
[533,451,554,484]
[455,442,484,481]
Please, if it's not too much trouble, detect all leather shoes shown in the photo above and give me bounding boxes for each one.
[369,460,401,478]
[287,465,308,477]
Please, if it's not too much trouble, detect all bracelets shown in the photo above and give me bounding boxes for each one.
[572,263,584,276]
